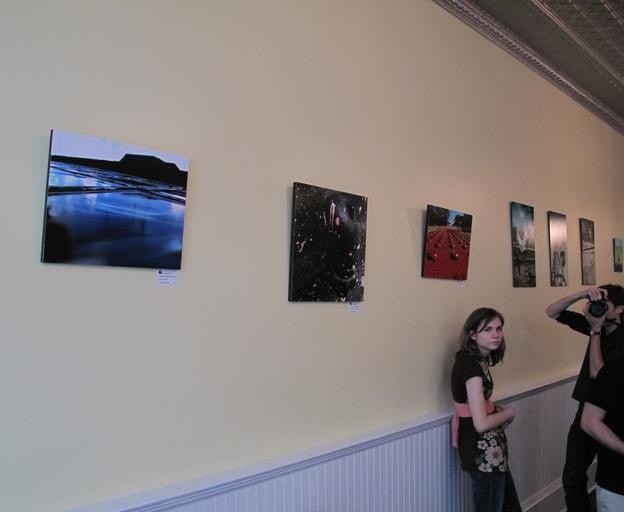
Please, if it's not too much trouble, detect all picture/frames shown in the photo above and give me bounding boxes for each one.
[509,201,539,288]
[613,238,624,272]
[288,182,367,301]
[548,212,569,287]
[421,204,472,281]
[41,129,188,271]
[579,217,596,286]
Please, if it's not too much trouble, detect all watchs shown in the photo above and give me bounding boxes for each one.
[589,330,601,336]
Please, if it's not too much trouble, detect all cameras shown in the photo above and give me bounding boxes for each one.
[587,292,609,319]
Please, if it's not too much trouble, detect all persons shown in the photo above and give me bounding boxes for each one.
[545,285,624,512]
[580,328,624,512]
[450,308,522,512]
